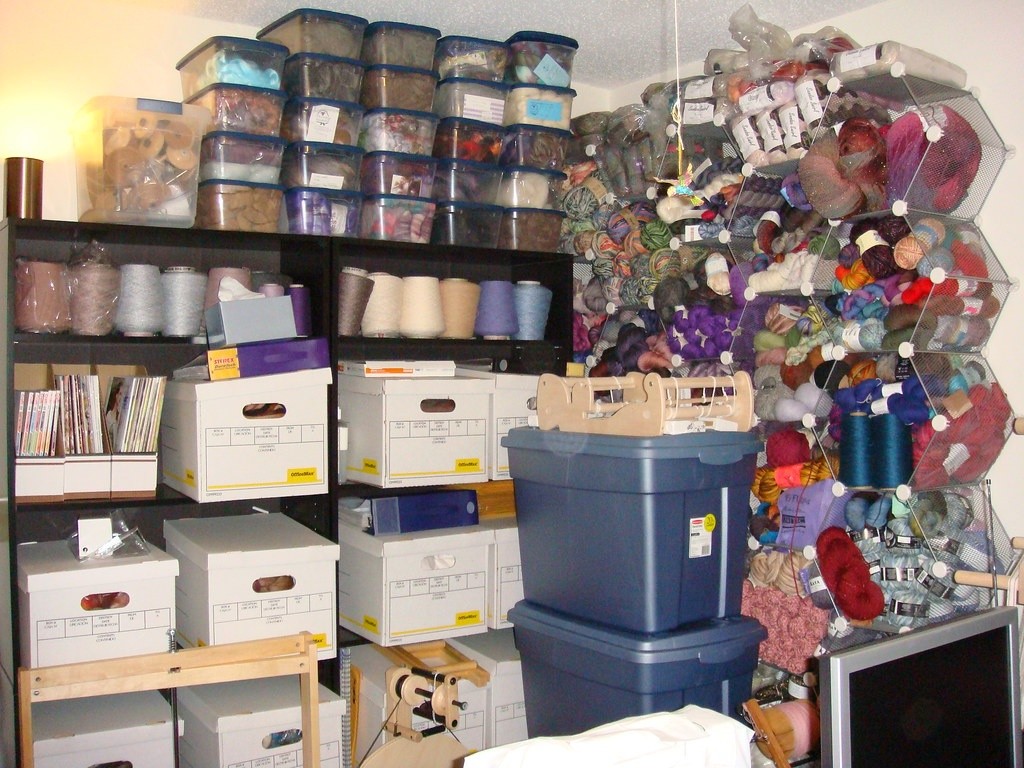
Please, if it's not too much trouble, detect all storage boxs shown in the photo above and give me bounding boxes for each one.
[13,511,526,768]
[73,8,578,254]
[501,424,769,739]
[158,368,540,504]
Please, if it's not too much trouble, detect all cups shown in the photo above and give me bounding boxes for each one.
[4,157,44,220]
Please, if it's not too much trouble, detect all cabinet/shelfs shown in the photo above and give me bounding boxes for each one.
[0,220,572,768]
[565,41,1016,634]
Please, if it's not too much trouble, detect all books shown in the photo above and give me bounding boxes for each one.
[13,375,167,458]
